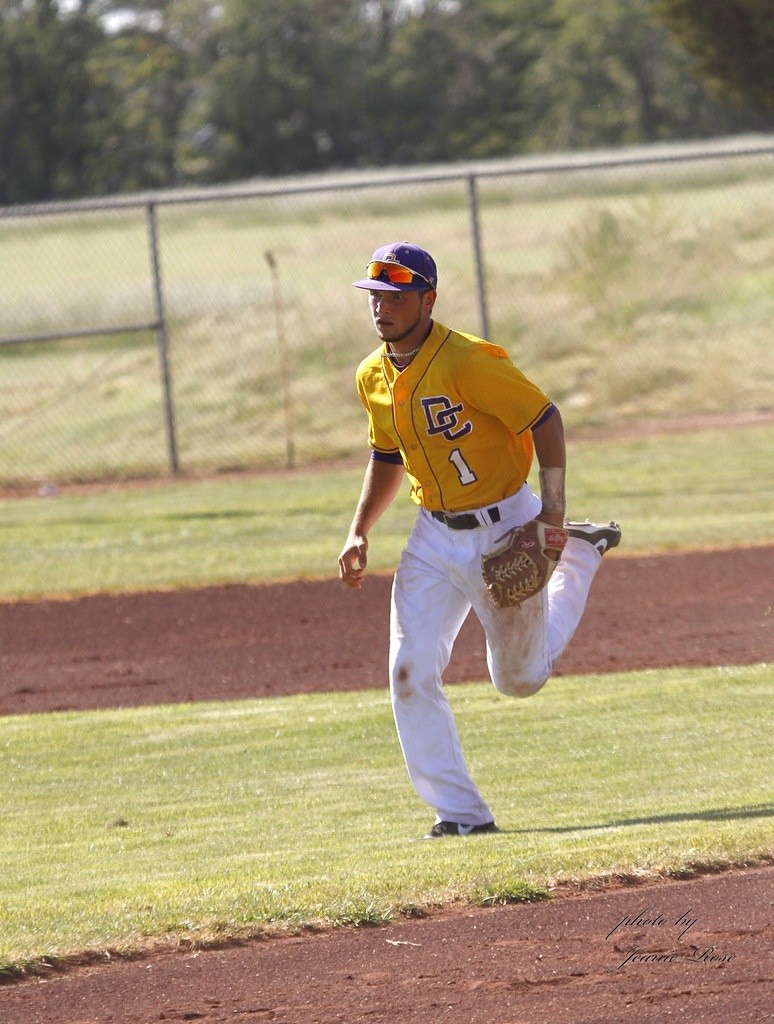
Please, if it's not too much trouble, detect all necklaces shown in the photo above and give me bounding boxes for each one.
[390,351,410,368]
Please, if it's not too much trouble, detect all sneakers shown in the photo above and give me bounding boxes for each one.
[564,518,622,556]
[432,820,494,836]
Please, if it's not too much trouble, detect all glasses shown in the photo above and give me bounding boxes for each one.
[365,260,435,289]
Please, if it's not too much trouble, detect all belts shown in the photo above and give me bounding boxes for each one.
[431,507,501,531]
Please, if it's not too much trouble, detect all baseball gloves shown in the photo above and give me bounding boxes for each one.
[480,514,574,610]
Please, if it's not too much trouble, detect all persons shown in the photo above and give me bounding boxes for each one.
[337,244,621,836]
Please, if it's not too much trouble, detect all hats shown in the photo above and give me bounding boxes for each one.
[353,241,437,291]
